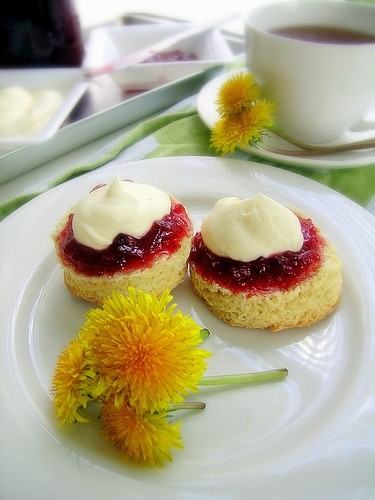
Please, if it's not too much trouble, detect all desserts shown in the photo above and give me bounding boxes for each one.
[188,192,343,330]
[53,178,194,303]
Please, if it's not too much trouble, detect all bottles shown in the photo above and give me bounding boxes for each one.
[1,1,84,67]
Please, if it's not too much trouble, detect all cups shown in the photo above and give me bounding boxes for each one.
[241,0,374,145]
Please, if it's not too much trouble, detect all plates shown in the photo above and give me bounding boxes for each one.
[196,67,374,170]
[1,152,375,500]
[0,66,95,158]
[88,23,234,90]
